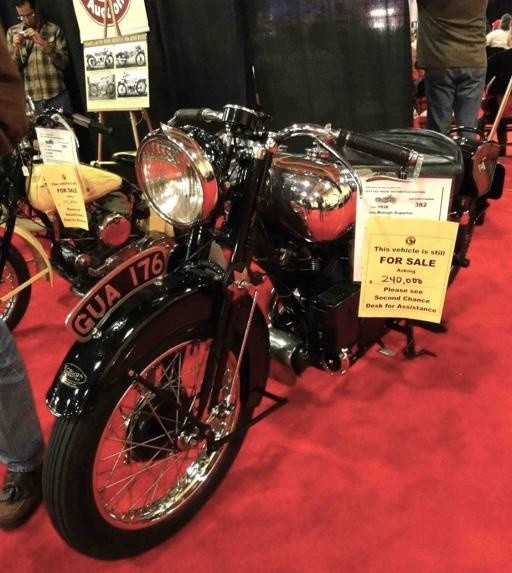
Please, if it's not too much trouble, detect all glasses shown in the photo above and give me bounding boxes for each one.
[16,9,36,19]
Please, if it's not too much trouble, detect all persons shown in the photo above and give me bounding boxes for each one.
[0,26,47,530]
[7,0,72,113]
[485,13,512,49]
[415,1,488,141]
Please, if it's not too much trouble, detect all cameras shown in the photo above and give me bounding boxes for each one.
[18,31,31,39]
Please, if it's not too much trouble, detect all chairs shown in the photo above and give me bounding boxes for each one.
[478,73,512,159]
[485,45,508,60]
[416,75,456,130]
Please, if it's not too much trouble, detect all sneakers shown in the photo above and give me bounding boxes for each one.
[0,464,42,529]
